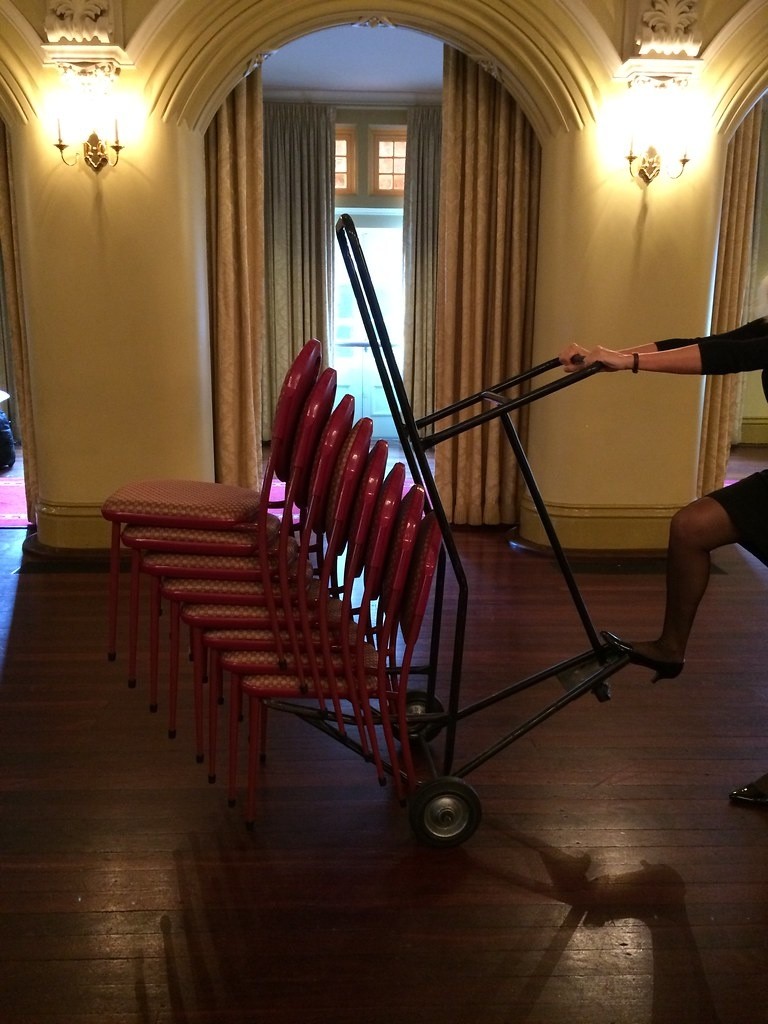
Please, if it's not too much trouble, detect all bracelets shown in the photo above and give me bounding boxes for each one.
[632,352,639,373]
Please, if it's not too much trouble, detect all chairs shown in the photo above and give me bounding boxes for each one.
[96,336,443,834]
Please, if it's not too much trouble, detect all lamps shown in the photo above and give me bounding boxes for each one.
[39,66,150,179]
[595,75,717,194]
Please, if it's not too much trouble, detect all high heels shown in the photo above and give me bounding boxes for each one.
[602,631,686,683]
[728,782,768,808]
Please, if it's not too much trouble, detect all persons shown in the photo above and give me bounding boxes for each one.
[560,272,768,809]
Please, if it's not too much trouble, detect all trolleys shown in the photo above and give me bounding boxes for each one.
[258,212,636,851]
